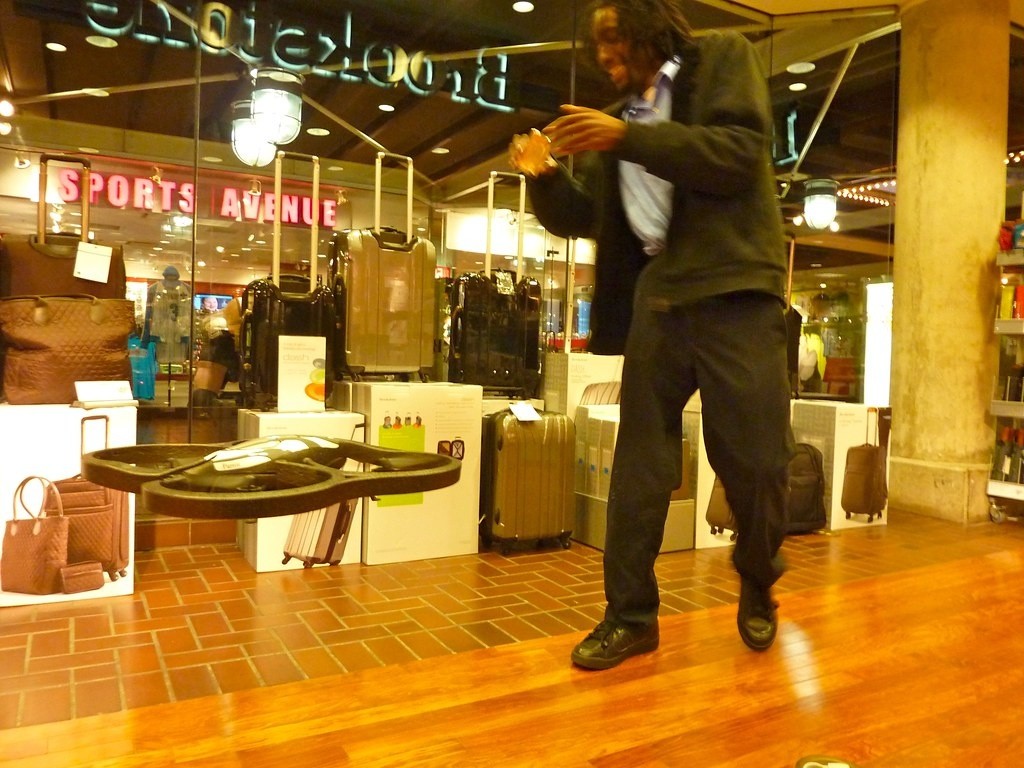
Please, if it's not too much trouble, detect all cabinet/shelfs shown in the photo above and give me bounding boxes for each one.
[986,246,1024,524]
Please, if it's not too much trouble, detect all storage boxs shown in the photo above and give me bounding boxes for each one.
[235,407,335,553]
[333,380,354,412]
[246,383,484,573]
[575,405,621,500]
[573,491,695,555]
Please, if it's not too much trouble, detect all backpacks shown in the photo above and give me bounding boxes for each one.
[786,441,828,534]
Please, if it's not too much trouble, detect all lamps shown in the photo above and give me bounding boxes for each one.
[243,58,306,146]
[803,174,840,231]
[229,95,277,167]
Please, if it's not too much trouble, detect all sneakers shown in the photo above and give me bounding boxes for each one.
[738,575,777,650]
[571,619,659,669]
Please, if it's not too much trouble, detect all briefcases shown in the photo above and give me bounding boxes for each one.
[238,151,346,409]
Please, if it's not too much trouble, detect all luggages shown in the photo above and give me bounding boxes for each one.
[782,228,801,399]
[449,173,542,401]
[481,407,571,555]
[841,406,888,521]
[0,152,127,300]
[328,152,440,383]
[284,423,366,568]
[46,416,129,582]
[705,473,739,541]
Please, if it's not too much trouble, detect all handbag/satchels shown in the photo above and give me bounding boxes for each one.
[59,558,105,593]
[125,333,157,401]
[0,293,134,405]
[1,475,69,595]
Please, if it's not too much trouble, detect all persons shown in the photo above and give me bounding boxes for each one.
[196,298,220,313]
[510,0,798,670]
[811,290,863,397]
